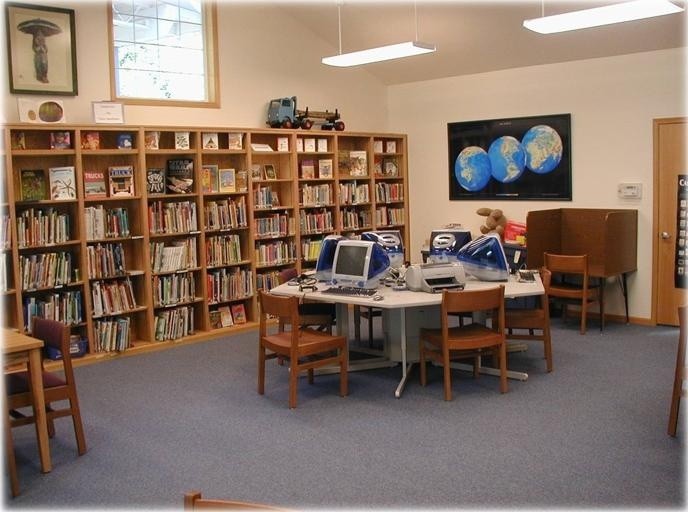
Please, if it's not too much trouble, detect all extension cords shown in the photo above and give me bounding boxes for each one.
[395,278,407,290]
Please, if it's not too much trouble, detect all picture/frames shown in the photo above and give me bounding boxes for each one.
[6,1,78,97]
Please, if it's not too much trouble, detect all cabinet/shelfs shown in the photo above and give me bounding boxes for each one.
[1,122,411,377]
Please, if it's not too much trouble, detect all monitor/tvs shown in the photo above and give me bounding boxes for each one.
[457,234,509,281]
[361,230,404,267]
[331,240,391,289]
[430,230,472,263]
[315,235,349,281]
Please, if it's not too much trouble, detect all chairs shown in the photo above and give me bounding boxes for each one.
[276,269,337,366]
[502,266,552,373]
[256,287,348,408]
[183,491,290,511]
[419,284,510,402]
[7,317,88,457]
[667,305,688,437]
[543,253,604,337]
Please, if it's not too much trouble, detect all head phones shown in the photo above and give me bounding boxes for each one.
[297,274,317,285]
[299,285,318,292]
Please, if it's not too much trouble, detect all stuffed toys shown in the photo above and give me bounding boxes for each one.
[474,207,506,238]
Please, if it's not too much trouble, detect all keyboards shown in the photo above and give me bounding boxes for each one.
[288,273,317,286]
[517,270,536,283]
[321,287,378,297]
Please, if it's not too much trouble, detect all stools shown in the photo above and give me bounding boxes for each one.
[351,303,386,348]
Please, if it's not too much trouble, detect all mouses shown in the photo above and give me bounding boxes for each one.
[374,294,384,301]
[531,270,538,274]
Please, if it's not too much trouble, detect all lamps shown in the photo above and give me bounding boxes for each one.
[521,0,684,36]
[321,0,437,67]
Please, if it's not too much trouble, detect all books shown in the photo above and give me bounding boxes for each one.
[3,127,136,359]
[145,131,254,343]
[339,140,404,229]
[251,138,333,321]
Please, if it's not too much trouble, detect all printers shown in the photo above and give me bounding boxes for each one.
[405,262,466,293]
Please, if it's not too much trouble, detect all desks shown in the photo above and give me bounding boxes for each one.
[3,330,51,474]
[525,208,638,336]
[269,262,546,397]
